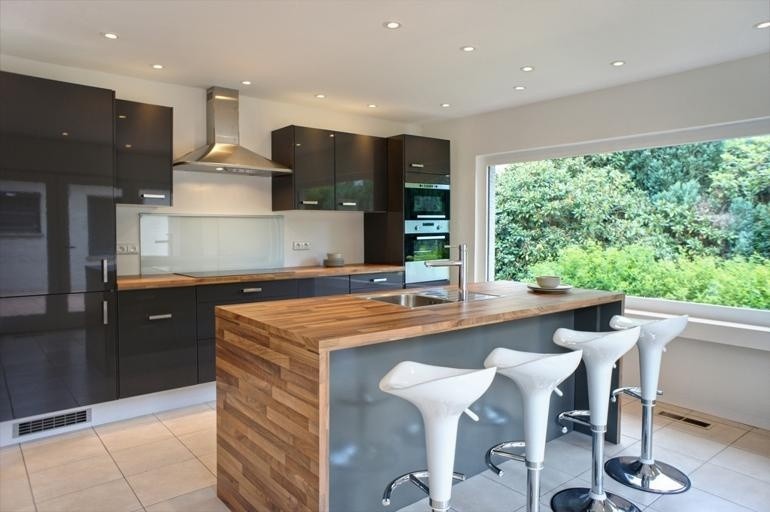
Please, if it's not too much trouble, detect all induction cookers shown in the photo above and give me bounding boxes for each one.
[174,270,296,279]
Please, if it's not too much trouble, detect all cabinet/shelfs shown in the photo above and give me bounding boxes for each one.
[388,134,450,183]
[195,277,300,384]
[299,273,350,298]
[1,71,120,421]
[271,125,388,212]
[349,269,403,295]
[115,99,176,208]
[120,286,200,400]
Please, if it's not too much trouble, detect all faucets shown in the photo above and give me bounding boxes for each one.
[424,243,469,302]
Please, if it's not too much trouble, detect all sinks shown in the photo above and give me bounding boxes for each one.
[368,294,452,308]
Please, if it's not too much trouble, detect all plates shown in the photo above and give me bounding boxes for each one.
[324,259,345,268]
[527,285,574,293]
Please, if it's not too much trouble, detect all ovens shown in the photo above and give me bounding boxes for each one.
[404,183,450,284]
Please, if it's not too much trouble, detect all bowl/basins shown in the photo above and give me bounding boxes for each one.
[536,275,561,288]
[327,253,343,260]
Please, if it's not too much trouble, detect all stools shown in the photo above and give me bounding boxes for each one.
[483,347,584,512]
[550,326,643,512]
[378,360,500,511]
[603,314,693,494]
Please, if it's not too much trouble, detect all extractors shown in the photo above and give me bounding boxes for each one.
[175,84,289,177]
